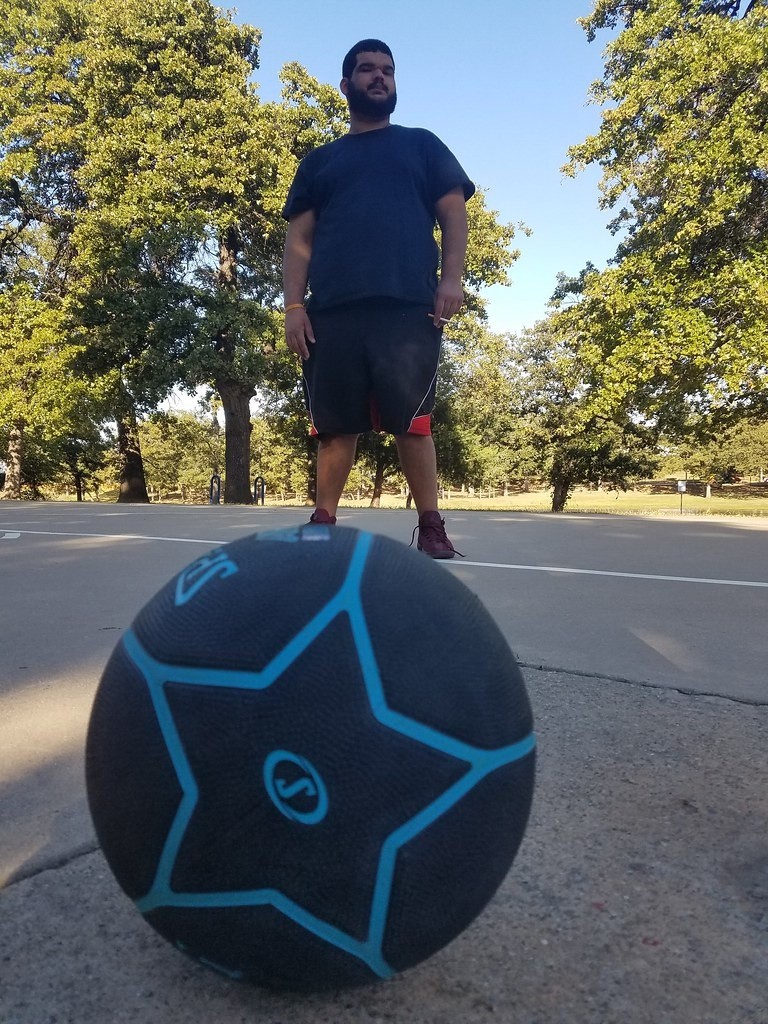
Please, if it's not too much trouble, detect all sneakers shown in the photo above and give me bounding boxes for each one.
[303,508,337,526]
[407,511,467,559]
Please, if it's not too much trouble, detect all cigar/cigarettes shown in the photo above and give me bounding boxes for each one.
[428,313,451,323]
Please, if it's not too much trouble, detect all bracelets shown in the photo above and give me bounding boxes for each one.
[284,303,303,313]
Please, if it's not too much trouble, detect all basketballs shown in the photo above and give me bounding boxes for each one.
[86,520,540,998]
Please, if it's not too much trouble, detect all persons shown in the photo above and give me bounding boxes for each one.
[281,39,475,559]
